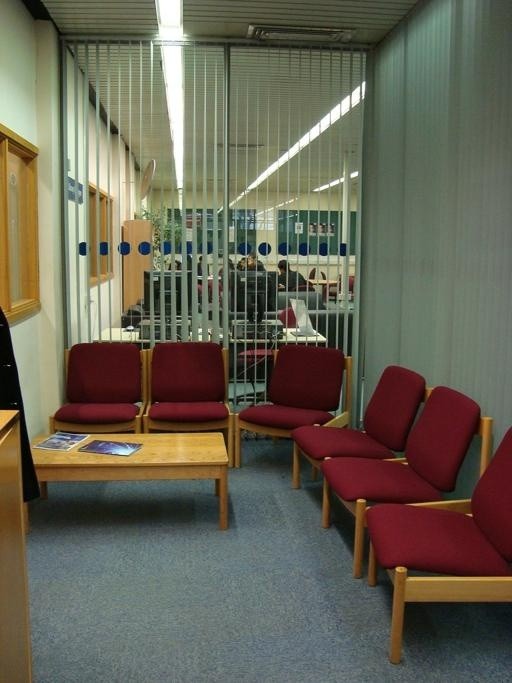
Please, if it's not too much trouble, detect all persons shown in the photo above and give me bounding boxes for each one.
[237,253,266,271]
[278,259,308,291]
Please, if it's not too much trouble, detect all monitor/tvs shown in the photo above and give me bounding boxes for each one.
[229,270,279,324]
[143,270,192,322]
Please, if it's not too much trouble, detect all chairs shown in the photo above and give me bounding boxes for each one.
[292,364,436,528]
[144,344,235,466]
[235,345,354,492]
[368,423,510,661]
[323,386,495,580]
[50,343,146,433]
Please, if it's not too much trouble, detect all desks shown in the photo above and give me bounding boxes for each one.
[97,321,328,343]
[234,348,279,403]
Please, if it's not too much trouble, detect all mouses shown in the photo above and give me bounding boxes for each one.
[126,324,136,332]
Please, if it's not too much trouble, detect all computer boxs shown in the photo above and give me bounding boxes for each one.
[231,318,285,339]
[137,318,191,340]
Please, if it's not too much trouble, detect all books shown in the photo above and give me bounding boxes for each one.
[77,439,144,456]
[32,431,90,452]
